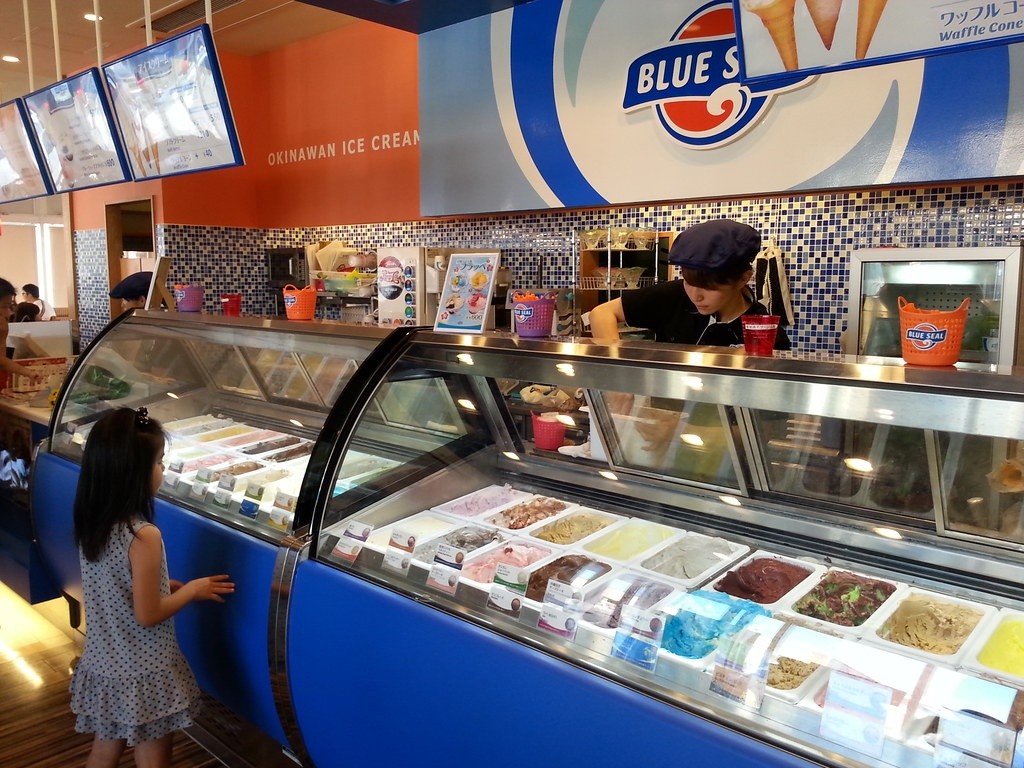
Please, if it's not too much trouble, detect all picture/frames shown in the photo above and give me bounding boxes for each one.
[432,253,501,335]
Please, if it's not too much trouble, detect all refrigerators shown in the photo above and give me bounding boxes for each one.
[374,246,438,327]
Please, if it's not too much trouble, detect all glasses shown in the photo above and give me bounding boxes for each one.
[22,292,24,294]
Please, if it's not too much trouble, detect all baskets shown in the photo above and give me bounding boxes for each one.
[512,288,556,337]
[898,296,971,366]
[282,283,317,320]
[173,281,204,312]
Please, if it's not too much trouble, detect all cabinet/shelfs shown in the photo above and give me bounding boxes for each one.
[580,231,659,340]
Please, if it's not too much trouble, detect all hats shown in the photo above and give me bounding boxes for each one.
[668,219,763,274]
[108,271,152,300]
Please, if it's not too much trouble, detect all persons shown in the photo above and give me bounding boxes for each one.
[109,271,154,313]
[67,406,238,767]
[1,278,59,395]
[588,218,794,478]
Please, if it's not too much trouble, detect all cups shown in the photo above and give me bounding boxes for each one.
[739,314,781,357]
[577,227,657,250]
[221,292,241,318]
[592,266,648,288]
[434,256,446,272]
